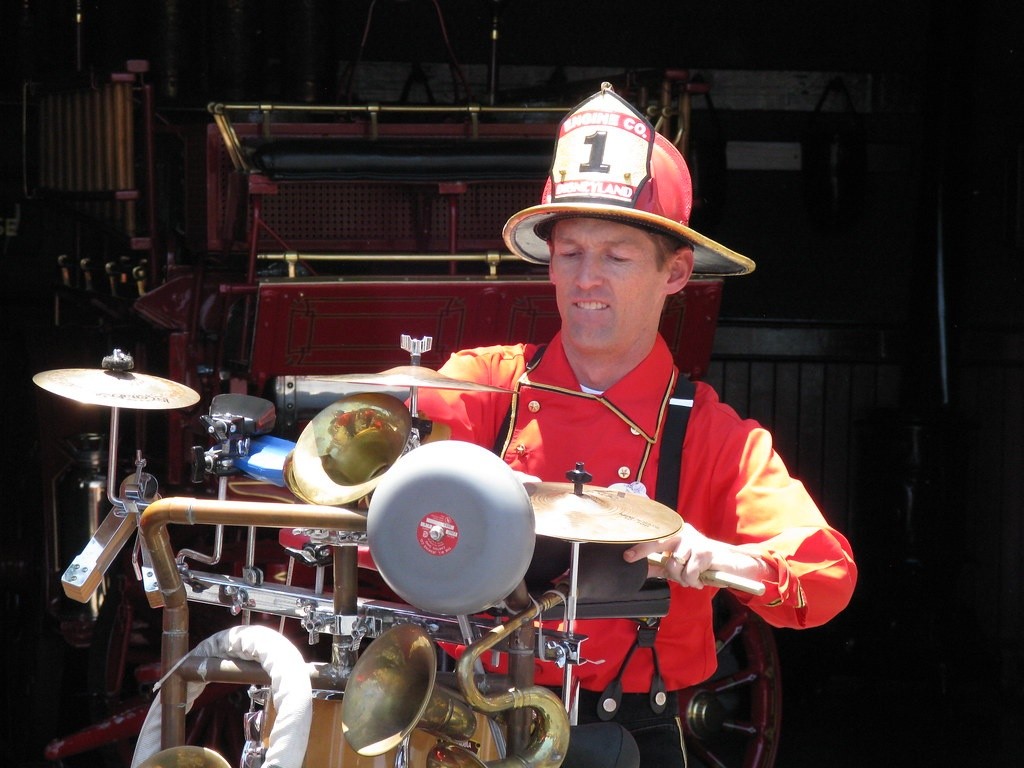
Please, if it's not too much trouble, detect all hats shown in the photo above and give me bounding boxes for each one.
[502,81,756,276]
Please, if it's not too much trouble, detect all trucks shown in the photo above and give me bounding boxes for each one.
[41,98,724,767]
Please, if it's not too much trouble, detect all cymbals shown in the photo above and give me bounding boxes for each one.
[296,332,521,398]
[30,345,203,414]
[521,460,687,547]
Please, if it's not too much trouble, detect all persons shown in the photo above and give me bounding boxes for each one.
[282,82,860,768]
